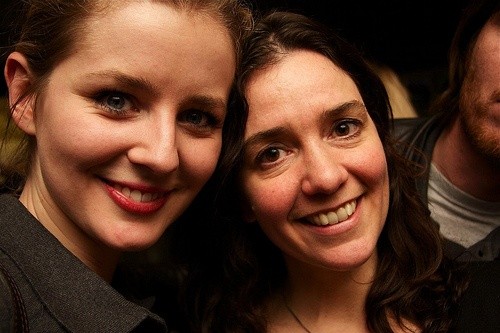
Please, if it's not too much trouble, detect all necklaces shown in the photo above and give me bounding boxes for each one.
[279,286,314,333]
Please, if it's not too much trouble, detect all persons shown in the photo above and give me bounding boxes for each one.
[224,9,499,333]
[378,1,500,271]
[0,0,259,333]
